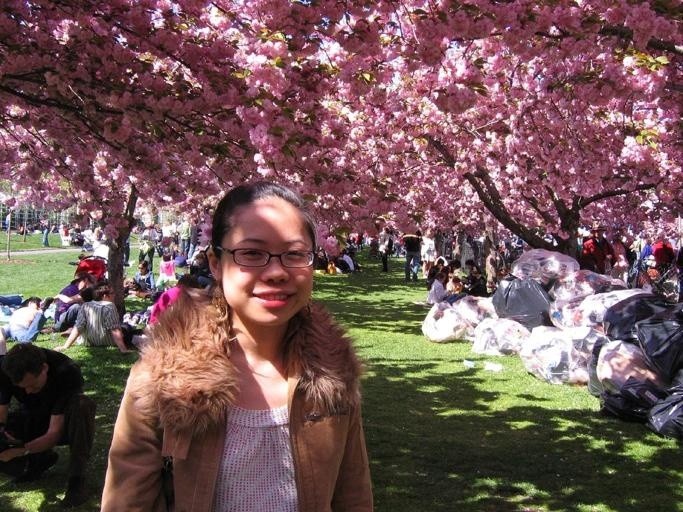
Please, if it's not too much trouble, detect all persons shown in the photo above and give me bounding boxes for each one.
[102,182,374,512]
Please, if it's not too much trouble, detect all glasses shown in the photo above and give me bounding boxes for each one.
[214,242,316,269]
